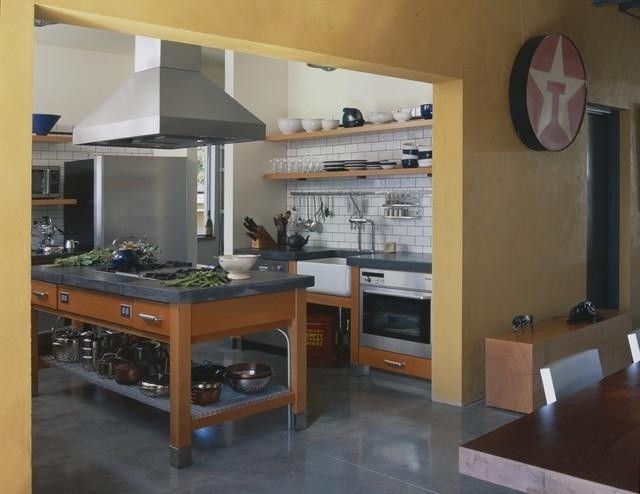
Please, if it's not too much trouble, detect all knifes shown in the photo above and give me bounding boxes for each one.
[243,216,257,240]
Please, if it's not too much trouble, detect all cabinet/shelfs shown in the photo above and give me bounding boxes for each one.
[261,117,432,182]
[31,134,81,206]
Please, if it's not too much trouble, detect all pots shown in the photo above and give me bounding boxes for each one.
[51,328,170,398]
[219,361,274,395]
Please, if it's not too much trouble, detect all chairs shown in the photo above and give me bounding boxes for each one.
[627,333,640,363]
[539,348,606,406]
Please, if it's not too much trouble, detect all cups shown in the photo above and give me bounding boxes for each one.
[269,157,323,173]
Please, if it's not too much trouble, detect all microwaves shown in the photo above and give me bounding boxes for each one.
[31,165,61,199]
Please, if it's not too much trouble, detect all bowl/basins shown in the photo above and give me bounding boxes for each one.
[33,113,62,135]
[393,110,410,123]
[189,382,222,405]
[217,253,261,279]
[380,162,396,169]
[368,113,390,126]
[420,104,431,119]
[322,119,340,130]
[401,144,419,168]
[300,119,326,133]
[277,118,302,136]
[418,145,432,167]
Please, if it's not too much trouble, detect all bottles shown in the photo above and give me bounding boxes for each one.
[384,208,407,217]
[206,210,214,235]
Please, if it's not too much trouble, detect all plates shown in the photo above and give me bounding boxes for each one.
[365,161,379,170]
[345,160,368,170]
[323,161,344,172]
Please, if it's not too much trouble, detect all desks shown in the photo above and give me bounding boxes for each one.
[457,362,639,494]
[485,308,633,415]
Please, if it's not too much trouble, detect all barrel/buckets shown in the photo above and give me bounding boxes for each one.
[306,309,340,369]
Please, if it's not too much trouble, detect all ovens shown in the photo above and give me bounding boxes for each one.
[358,267,431,359]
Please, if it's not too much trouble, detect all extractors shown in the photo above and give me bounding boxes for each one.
[73,34,266,150]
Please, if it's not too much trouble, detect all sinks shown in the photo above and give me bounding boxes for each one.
[296,257,352,298]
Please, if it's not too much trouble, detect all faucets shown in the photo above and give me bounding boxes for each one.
[349,217,376,253]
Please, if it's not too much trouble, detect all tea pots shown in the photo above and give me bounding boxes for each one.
[282,231,310,249]
